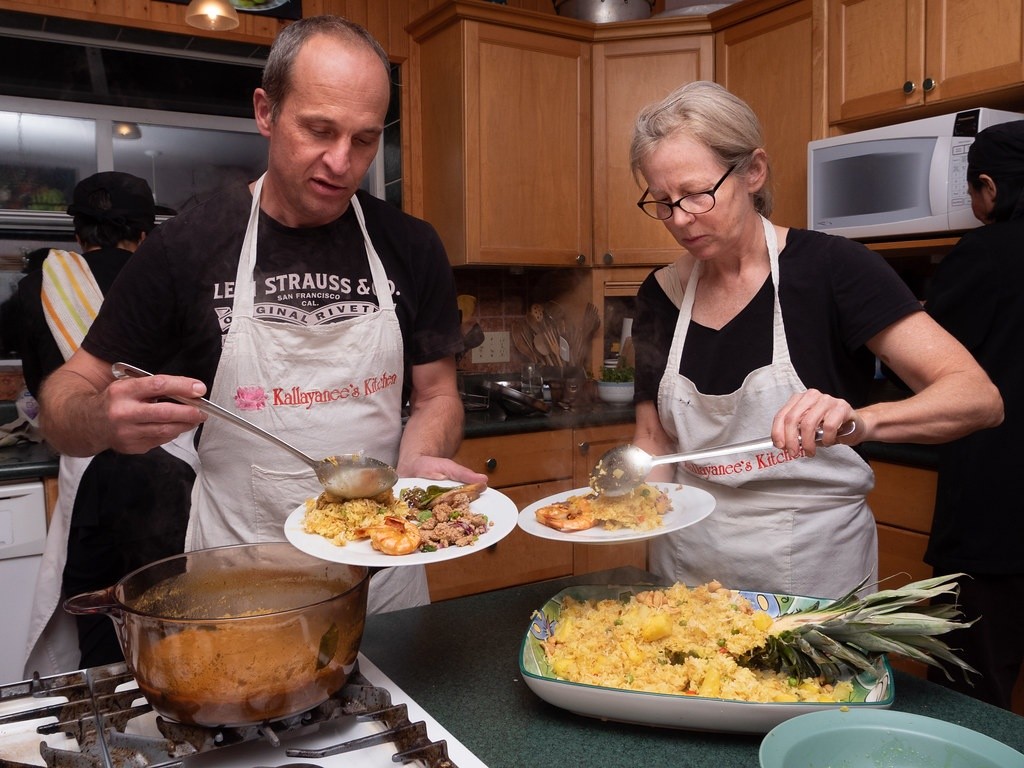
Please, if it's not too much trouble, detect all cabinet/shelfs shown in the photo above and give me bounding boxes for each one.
[429,420,644,601]
[864,463,938,679]
[405,0,1024,377]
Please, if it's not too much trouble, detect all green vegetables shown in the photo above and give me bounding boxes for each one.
[588,357,635,383]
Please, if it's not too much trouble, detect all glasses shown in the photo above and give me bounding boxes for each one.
[636,163,738,220]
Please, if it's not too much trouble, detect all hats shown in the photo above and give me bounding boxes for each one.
[66,171,179,216]
[967,120,1024,175]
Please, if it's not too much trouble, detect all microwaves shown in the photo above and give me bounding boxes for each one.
[806,105,1023,239]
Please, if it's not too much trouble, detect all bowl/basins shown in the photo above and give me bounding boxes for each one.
[594,380,637,405]
[757,708,1024,768]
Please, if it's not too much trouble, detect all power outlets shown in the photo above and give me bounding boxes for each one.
[470,332,511,364]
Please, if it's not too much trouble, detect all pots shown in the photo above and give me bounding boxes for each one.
[62,543,371,727]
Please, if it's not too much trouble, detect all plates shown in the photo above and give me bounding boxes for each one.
[283,477,519,567]
[517,480,719,543]
[517,584,904,732]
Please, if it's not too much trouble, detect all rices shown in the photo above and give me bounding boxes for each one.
[540,581,848,701]
[301,485,411,546]
[565,459,667,530]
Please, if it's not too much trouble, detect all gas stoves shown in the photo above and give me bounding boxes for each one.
[0,645,489,767]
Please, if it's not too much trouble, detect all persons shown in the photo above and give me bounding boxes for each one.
[881,121,1024,717]
[9,171,222,680]
[629,81,1004,601]
[39,14,488,616]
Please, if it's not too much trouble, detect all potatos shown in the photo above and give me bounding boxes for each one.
[550,608,838,703]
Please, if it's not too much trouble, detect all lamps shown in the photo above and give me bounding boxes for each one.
[111,122,141,141]
[185,0,239,31]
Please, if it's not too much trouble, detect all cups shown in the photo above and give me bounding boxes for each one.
[519,363,584,406]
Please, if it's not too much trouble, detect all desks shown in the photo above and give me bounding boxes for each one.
[365,567,1023,765]
